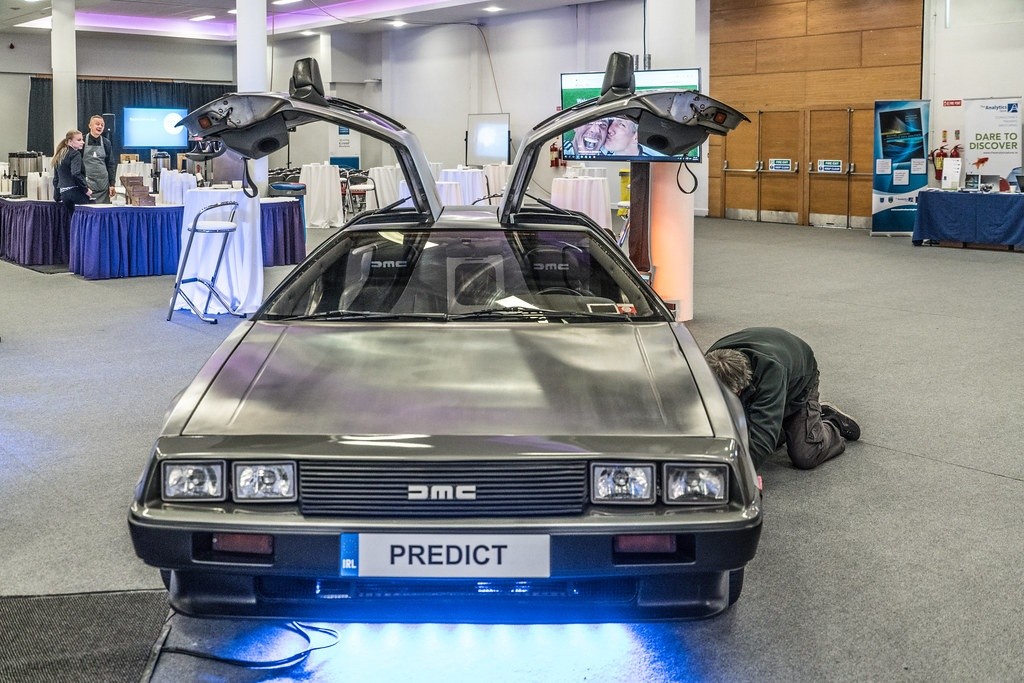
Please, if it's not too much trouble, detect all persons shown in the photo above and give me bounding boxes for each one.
[78,115,116,204]
[51,130,96,216]
[705,327,861,469]
[563,113,650,156]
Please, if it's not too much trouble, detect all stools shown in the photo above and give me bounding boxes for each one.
[165,201,248,324]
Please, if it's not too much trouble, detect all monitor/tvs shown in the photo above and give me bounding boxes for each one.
[120,106,189,149]
[559,67,703,163]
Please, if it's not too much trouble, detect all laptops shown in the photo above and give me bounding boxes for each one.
[1016,176,1024,193]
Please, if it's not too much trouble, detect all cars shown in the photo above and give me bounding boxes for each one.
[125,51,765,625]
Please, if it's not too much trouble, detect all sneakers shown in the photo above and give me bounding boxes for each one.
[820,402,861,441]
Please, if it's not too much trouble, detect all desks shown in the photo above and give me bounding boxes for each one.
[299,164,612,236]
[0,163,306,280]
[911,190,1023,253]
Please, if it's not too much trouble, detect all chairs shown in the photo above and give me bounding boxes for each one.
[339,248,436,313]
[618,188,631,246]
[505,248,582,301]
[268,163,380,221]
